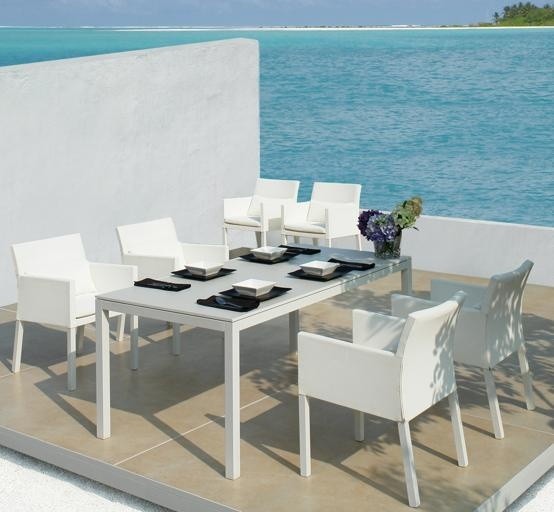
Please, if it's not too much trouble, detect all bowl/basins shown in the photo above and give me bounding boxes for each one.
[250,246,288,261]
[186,260,225,276]
[231,277,276,297]
[299,260,341,276]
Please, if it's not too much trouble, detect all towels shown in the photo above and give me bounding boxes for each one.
[278,244,322,256]
[327,258,376,272]
[196,294,261,314]
[132,276,193,292]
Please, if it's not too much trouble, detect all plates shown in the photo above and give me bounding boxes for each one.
[287,267,352,282]
[239,252,300,265]
[219,285,292,302]
[171,267,238,281]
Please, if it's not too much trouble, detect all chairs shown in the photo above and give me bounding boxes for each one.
[114,216,229,357]
[10,229,139,395]
[293,287,472,509]
[222,178,299,248]
[280,181,362,251]
[388,259,538,442]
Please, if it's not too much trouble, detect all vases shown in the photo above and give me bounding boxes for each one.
[373,232,401,260]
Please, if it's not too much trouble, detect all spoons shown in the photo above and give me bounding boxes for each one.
[214,296,243,311]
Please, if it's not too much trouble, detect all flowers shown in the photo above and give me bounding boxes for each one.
[356,194,424,259]
